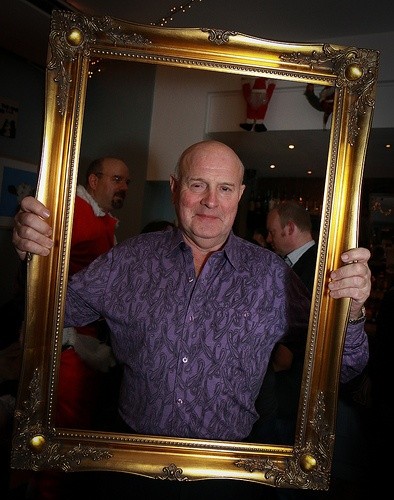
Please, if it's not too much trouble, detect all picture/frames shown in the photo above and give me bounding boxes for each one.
[10,8,382,491]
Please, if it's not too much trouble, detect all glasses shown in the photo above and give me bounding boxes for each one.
[97,173,133,185]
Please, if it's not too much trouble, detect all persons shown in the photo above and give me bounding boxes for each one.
[140,202,325,400]
[12,139,372,441]
[49,155,131,429]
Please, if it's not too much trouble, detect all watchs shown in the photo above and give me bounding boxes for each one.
[346,307,368,325]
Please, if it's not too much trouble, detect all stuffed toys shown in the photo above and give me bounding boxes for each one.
[239,77,275,132]
